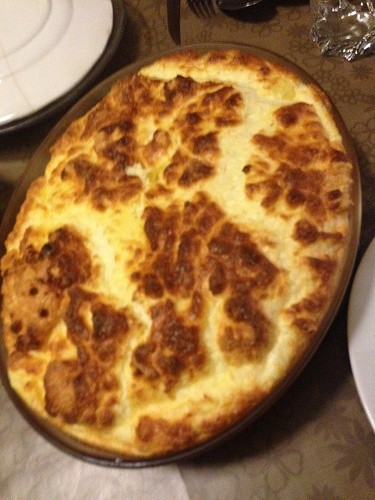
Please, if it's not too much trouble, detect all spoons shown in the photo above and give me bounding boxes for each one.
[217,0,260,10]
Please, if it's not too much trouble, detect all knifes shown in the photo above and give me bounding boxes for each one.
[167,0,181,45]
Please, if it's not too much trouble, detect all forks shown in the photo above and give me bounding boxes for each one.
[186,0,216,18]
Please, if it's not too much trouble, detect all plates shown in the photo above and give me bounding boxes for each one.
[0,0,128,134]
[1,41,361,469]
[346,237,375,432]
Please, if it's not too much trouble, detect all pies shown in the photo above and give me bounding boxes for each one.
[0,51,362,460]
